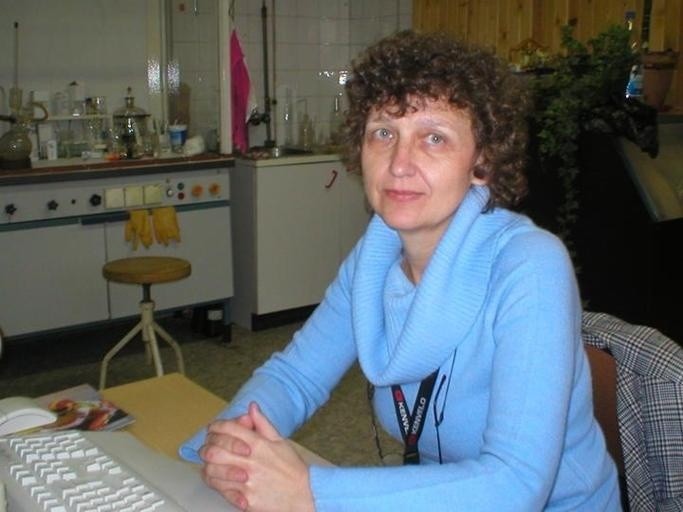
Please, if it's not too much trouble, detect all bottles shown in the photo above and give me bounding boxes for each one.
[329,96,344,145]
[115,96,152,157]
[299,113,314,150]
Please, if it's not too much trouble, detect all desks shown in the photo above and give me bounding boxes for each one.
[98,374,341,469]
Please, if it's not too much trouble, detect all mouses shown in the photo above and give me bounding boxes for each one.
[0,394,57,436]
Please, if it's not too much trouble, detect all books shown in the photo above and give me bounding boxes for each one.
[4,381,136,440]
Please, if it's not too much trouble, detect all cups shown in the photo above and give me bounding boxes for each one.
[167,125,187,152]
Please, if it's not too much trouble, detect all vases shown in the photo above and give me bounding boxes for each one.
[636,53,683,116]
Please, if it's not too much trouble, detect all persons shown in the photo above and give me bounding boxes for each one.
[173,29,625,512]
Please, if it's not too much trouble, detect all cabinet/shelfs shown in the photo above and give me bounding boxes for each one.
[0,161,370,346]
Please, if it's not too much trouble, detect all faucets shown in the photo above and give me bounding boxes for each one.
[250,112,270,126]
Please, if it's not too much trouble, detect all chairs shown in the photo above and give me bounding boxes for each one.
[583,344,623,478]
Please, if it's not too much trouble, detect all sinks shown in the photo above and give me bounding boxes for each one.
[248,149,313,157]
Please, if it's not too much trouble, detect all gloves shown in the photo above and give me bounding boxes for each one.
[124,208,153,250]
[152,207,180,245]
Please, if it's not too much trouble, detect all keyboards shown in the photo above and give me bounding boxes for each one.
[0,427,255,512]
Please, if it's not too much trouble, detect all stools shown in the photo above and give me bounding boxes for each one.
[99,256,192,387]
